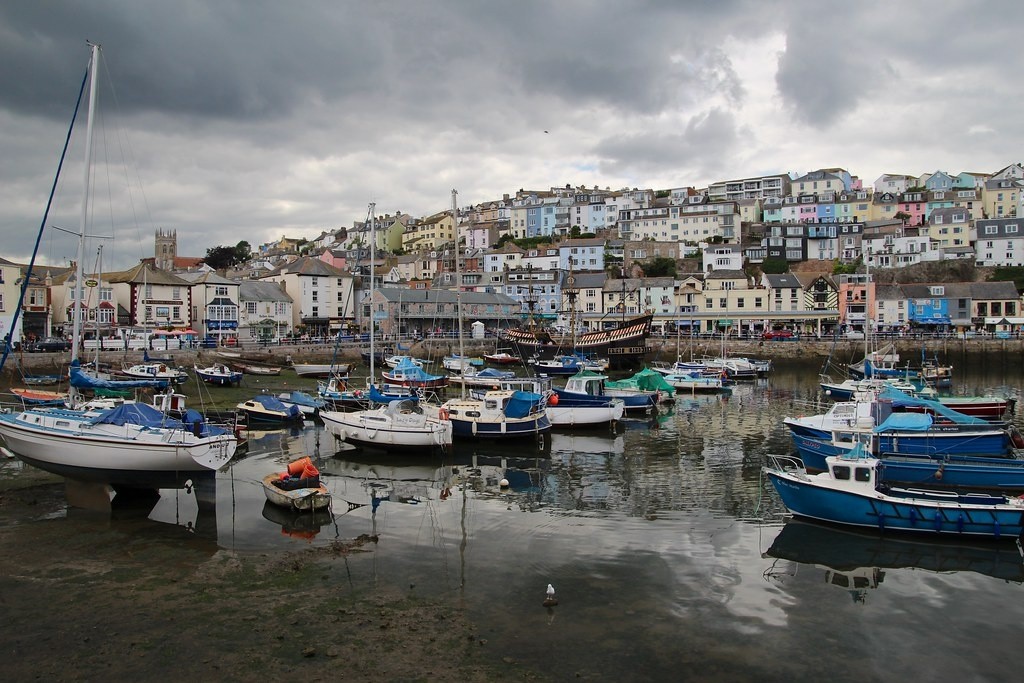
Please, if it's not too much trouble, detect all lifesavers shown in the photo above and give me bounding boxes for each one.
[357,390,365,399]
[74,396,82,402]
[439,408,450,420]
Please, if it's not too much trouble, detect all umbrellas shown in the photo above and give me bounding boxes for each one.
[184,330,198,334]
[170,330,186,335]
[154,330,172,336]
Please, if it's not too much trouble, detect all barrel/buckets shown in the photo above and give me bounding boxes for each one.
[288,456,319,480]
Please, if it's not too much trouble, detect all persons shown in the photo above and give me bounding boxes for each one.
[4,333,9,340]
[287,330,292,338]
[99,333,103,351]
[436,326,441,338]
[286,353,291,367]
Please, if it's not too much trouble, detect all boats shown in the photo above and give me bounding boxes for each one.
[552,369,659,409]
[851,382,1007,419]
[782,431,1024,486]
[317,375,370,400]
[482,352,519,363]
[262,457,331,512]
[293,363,354,376]
[275,391,330,415]
[193,362,243,385]
[840,343,952,387]
[121,361,189,384]
[761,442,1024,537]
[782,384,1011,458]
[463,366,515,379]
[382,356,445,387]
[546,398,624,425]
[819,378,939,402]
[531,349,604,375]
[385,355,424,370]
[232,363,281,375]
[237,394,302,423]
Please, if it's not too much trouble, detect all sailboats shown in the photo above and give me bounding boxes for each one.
[440,188,553,438]
[0,39,238,471]
[662,283,773,390]
[319,201,453,448]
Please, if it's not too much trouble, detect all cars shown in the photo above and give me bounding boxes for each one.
[762,329,792,341]
